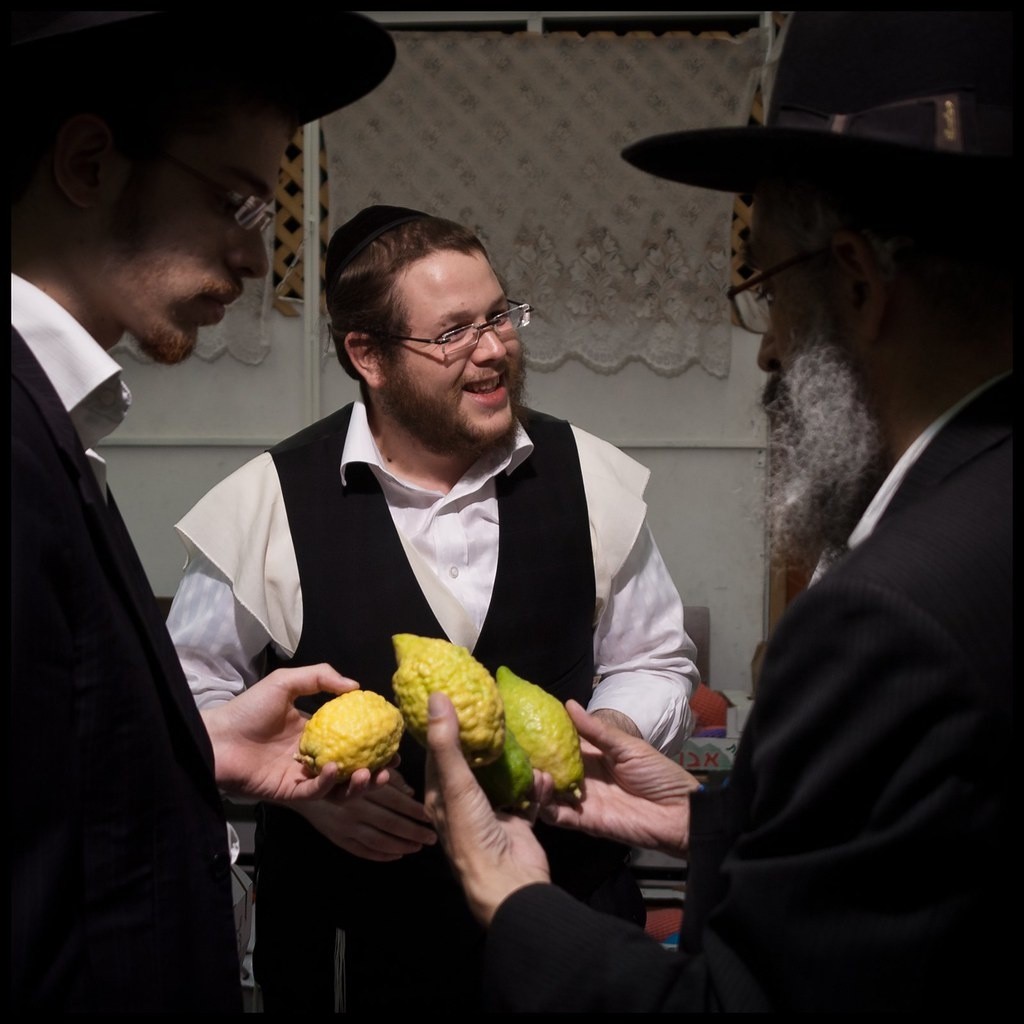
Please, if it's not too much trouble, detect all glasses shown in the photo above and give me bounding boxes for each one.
[727,241,838,334]
[111,123,274,235]
[371,298,535,356]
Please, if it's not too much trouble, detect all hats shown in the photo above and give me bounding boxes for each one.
[621,8,1024,198]
[0,0,397,130]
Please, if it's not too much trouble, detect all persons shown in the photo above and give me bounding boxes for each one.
[425,1,1024,1024]
[2,4,399,1024]
[163,203,647,1024]
